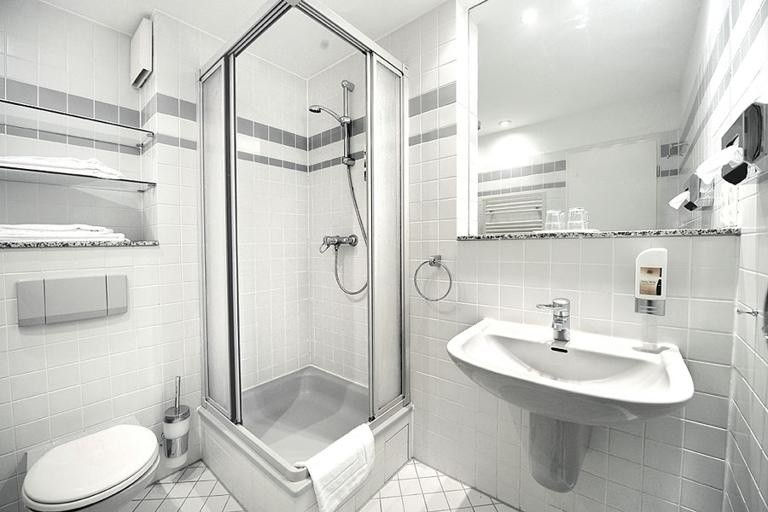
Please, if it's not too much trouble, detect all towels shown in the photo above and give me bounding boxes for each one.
[0,223,132,247]
[0,153,125,181]
[296,422,377,512]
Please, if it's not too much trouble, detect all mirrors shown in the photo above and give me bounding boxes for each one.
[454,0,743,241]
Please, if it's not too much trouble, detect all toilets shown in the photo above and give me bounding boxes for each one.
[20,415,161,512]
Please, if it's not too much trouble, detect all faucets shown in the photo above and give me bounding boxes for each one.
[536,298,570,341]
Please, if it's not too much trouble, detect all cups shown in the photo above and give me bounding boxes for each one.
[575,210,590,231]
[552,213,565,230]
[545,210,560,232]
[566,207,584,232]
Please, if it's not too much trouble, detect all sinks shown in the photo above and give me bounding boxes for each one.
[446,318,696,427]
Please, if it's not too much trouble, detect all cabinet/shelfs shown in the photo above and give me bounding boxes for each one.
[0,100,158,251]
